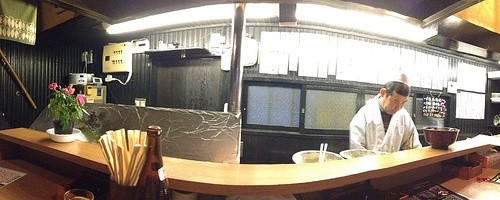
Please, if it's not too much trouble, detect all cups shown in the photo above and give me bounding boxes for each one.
[64,189,94,200]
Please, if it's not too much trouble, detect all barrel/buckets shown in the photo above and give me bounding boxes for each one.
[109,175,139,200]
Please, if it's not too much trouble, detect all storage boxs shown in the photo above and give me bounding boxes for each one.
[448,161,483,180]
[470,153,500,168]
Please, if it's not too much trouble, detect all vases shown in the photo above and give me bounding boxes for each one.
[51,119,73,134]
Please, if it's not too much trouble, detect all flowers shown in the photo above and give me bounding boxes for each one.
[47,79,90,130]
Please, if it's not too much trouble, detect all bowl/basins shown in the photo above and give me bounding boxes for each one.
[423,126,460,150]
[340,148,389,159]
[292,149,345,164]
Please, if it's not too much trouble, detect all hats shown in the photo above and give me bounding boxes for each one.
[386,71,410,87]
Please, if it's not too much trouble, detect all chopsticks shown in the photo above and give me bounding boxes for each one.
[98,128,148,186]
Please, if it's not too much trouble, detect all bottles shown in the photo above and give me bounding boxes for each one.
[140,125,169,200]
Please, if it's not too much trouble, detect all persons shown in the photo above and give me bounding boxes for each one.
[349,81,422,155]
